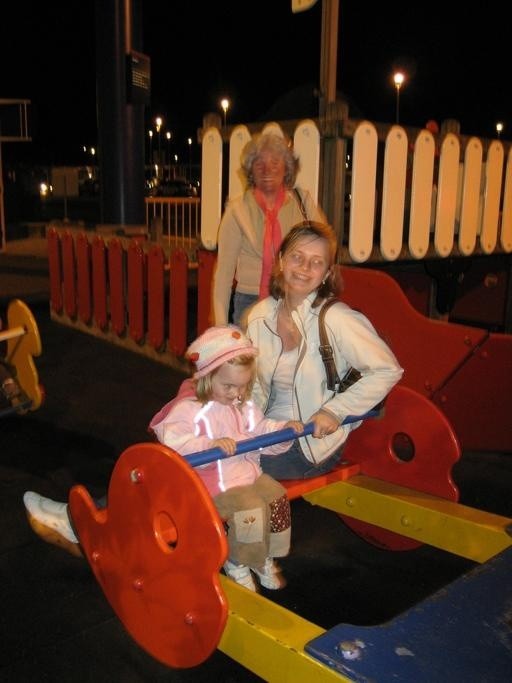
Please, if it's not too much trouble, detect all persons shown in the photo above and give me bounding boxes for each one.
[213,133,338,327]
[238,221,404,479]
[150,323,290,594]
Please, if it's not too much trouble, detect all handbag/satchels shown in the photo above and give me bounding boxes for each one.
[335,365,386,413]
[319,269,341,300]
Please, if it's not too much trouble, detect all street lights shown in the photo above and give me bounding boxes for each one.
[393,73,404,123]
[221,99,229,125]
[149,117,192,179]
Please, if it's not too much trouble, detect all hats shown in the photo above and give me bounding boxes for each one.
[184,323,258,380]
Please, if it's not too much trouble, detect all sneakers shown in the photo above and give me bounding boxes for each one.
[224,560,258,593]
[250,556,282,591]
